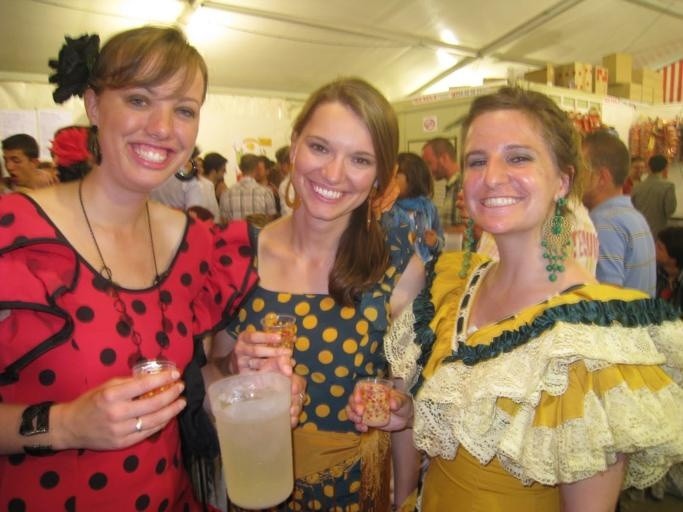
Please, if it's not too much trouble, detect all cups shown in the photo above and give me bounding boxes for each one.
[354,379,394,427]
[259,315,296,358]
[132,360,176,398]
[206,372,296,510]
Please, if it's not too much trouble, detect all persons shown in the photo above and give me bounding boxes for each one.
[459,132,683,304]
[375,137,471,273]
[176,77,443,512]
[345,85,682,512]
[1,25,260,512]
[149,146,296,226]
[1,126,93,195]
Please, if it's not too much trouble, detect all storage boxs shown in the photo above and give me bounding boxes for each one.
[520,47,664,106]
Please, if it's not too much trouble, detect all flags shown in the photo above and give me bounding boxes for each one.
[657,59,682,104]
